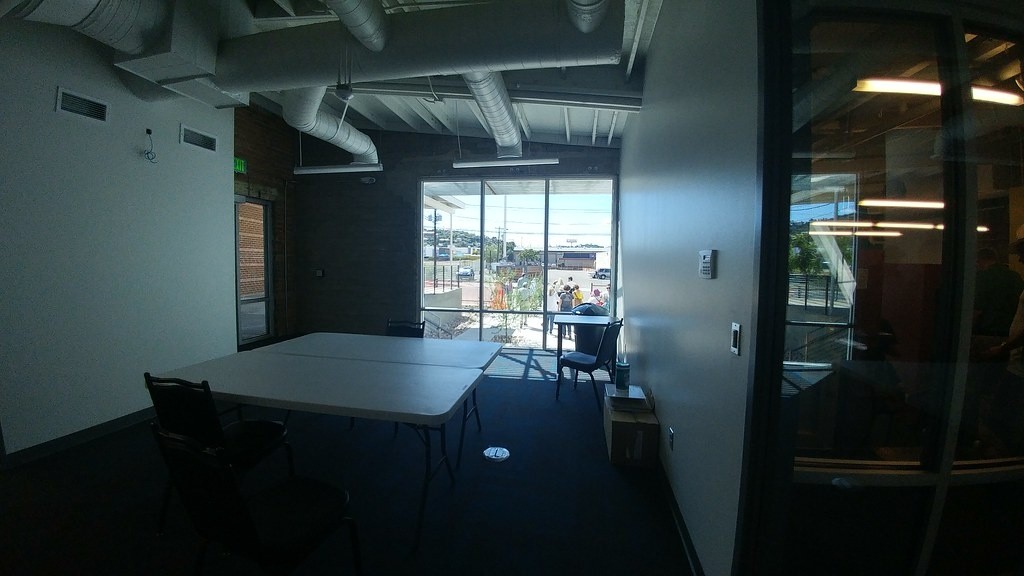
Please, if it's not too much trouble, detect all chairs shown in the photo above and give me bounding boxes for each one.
[556,318,624,413]
[349,318,425,438]
[148,421,362,576]
[144,372,296,539]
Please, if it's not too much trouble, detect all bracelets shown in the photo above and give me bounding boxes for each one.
[1000,342,1009,351]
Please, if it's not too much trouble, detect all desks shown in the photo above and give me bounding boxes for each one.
[786,314,857,329]
[554,314,624,376]
[156,332,502,559]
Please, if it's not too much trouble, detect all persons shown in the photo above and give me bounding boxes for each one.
[506,274,612,340]
[958,239,1024,452]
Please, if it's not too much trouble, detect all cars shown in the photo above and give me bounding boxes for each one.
[455,267,474,277]
[438,253,449,257]
[597,268,611,279]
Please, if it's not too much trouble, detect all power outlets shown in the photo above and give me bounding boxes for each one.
[668,428,674,451]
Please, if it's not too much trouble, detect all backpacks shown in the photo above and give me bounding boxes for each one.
[594,297,604,306]
[562,293,572,307]
[574,292,583,306]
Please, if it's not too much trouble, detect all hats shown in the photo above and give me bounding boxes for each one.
[594,289,601,293]
[522,282,529,287]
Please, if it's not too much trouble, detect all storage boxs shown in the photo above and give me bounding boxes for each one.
[603,399,660,468]
